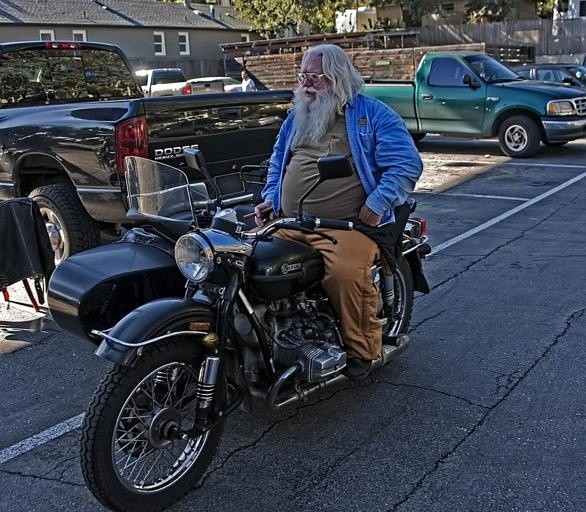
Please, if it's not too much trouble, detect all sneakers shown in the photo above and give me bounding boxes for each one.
[346,355,372,380]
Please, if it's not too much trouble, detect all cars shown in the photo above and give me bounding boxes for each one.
[502,58,586,86]
[189,74,243,95]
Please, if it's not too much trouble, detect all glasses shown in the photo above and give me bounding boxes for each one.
[297,72,325,83]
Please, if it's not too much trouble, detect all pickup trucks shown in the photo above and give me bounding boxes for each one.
[113,65,225,96]
[0,38,299,281]
[358,46,586,160]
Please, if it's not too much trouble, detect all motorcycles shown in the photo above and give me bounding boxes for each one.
[37,144,435,512]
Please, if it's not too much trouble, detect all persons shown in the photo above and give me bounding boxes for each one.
[245,42,424,380]
[241,70,258,92]
